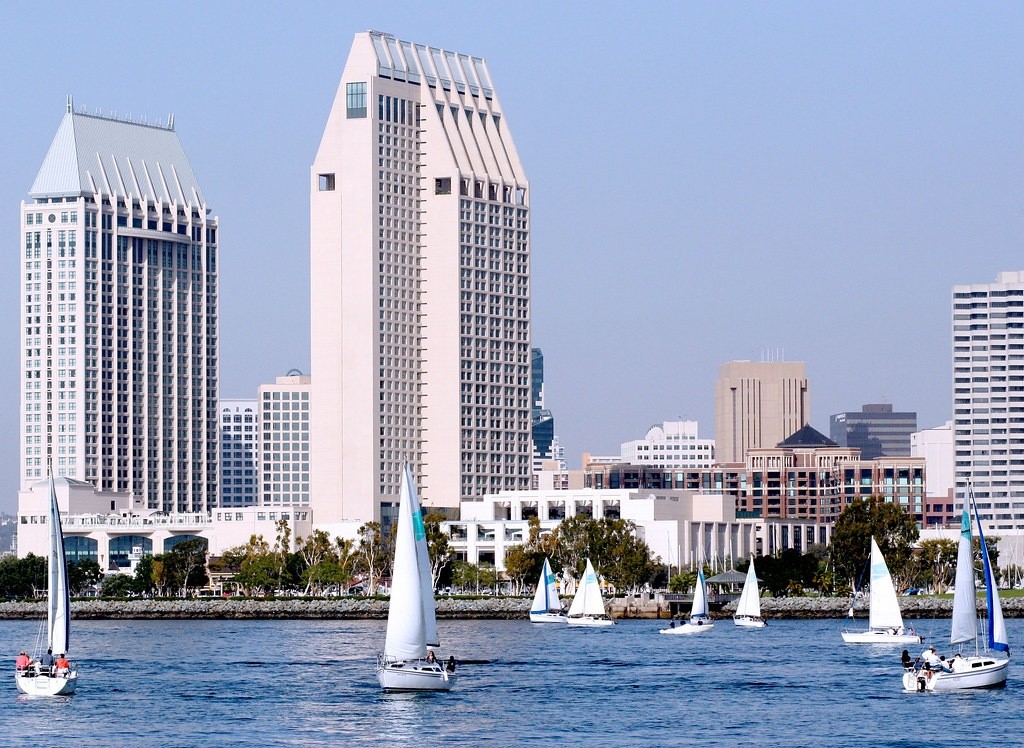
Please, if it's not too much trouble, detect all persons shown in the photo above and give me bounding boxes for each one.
[698,619,702,625]
[668,620,675,628]
[901,648,954,673]
[16,650,69,669]
[426,651,455,672]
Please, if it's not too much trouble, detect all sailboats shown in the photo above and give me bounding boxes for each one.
[659,560,717,635]
[529,556,567,625]
[902,478,1012,690]
[732,552,767,628]
[568,557,616,628]
[15,457,80,697]
[839,535,924,645]
[375,453,458,694]
